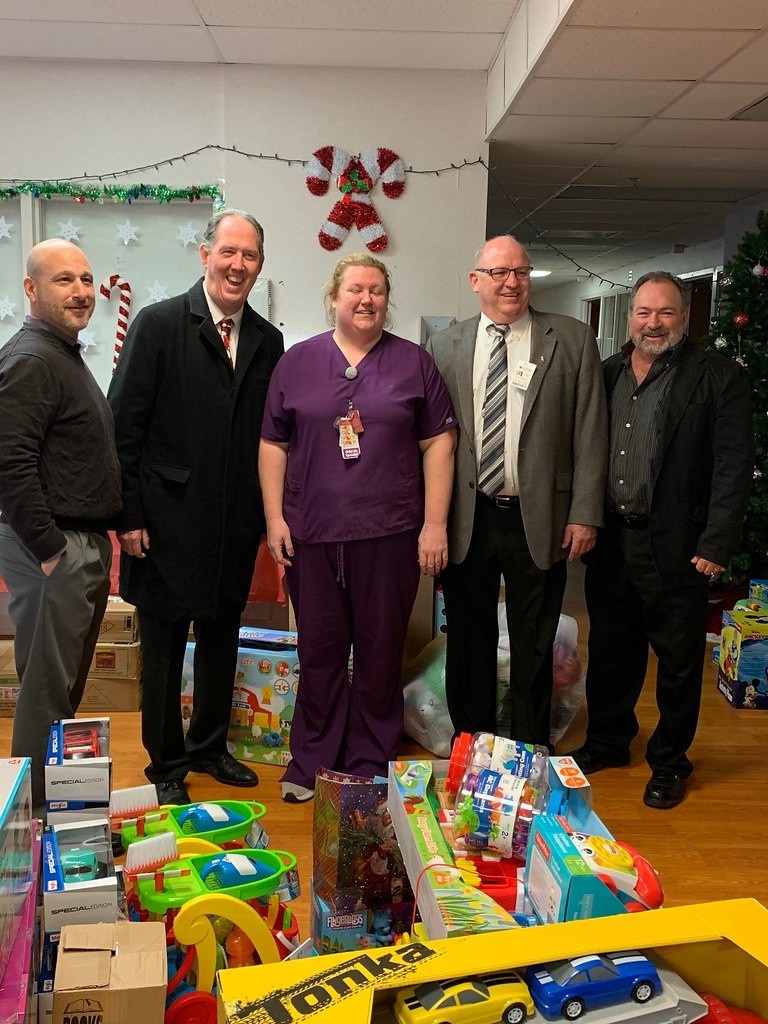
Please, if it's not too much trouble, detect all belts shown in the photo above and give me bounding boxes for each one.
[477,493,520,513]
[606,510,650,530]
[1,510,109,534]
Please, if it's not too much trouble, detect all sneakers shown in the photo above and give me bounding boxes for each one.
[281,781,315,801]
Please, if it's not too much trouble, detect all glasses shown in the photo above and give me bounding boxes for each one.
[475,266,534,281]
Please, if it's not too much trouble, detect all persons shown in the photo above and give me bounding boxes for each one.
[256,256,459,805]
[104,209,284,807]
[2,237,122,807]
[567,273,755,807]
[424,233,609,756]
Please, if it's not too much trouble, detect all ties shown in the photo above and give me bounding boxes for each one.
[220,319,233,368]
[478,323,510,500]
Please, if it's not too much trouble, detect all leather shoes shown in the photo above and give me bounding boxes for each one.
[155,779,191,806]
[643,770,688,810]
[565,746,632,774]
[190,752,258,786]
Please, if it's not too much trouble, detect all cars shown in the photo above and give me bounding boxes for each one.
[524,950,663,1023]
[395,970,535,1024]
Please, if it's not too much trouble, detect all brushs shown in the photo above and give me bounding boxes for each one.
[108,784,160,819]
[121,832,181,878]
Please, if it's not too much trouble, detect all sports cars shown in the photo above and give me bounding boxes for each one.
[58,849,99,883]
[62,728,101,762]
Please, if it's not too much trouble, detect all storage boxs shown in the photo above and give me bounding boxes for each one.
[0,639,21,718]
[96,599,137,642]
[52,920,168,1024]
[76,678,143,712]
[86,643,143,680]
